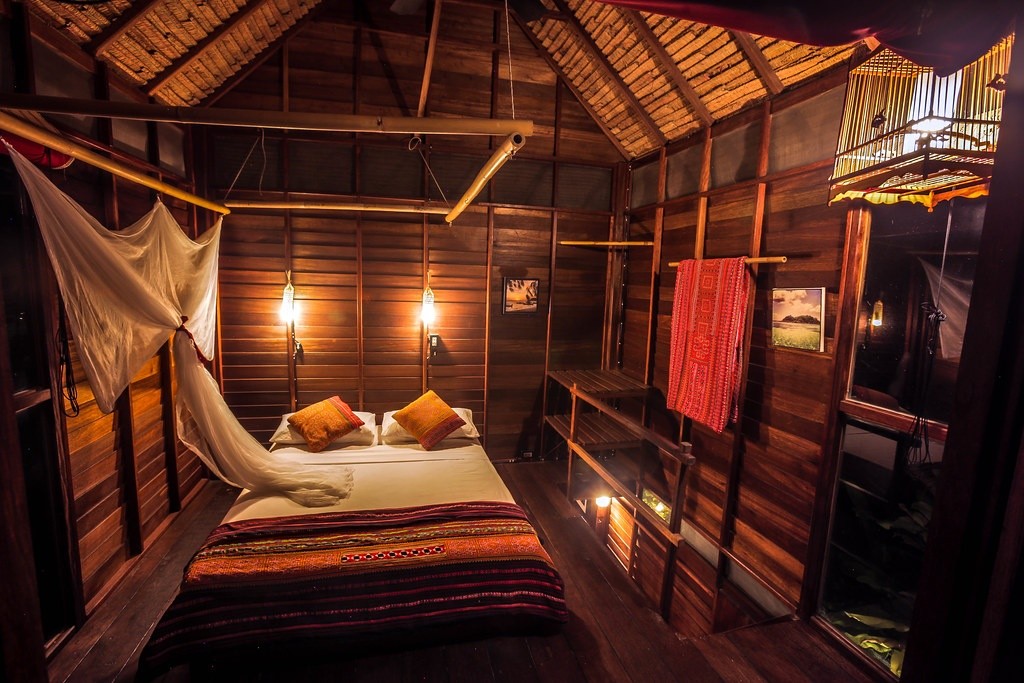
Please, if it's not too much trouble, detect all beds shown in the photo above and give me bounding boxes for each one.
[137,424,567,683]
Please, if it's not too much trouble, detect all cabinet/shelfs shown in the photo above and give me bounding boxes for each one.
[540,369,652,501]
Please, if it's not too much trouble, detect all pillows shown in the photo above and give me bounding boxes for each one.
[381,408,480,443]
[287,395,365,452]
[269,411,375,444]
[391,390,466,451]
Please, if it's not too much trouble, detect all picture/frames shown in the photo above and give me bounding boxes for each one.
[503,277,541,314]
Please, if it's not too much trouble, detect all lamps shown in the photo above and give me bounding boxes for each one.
[865,300,884,348]
